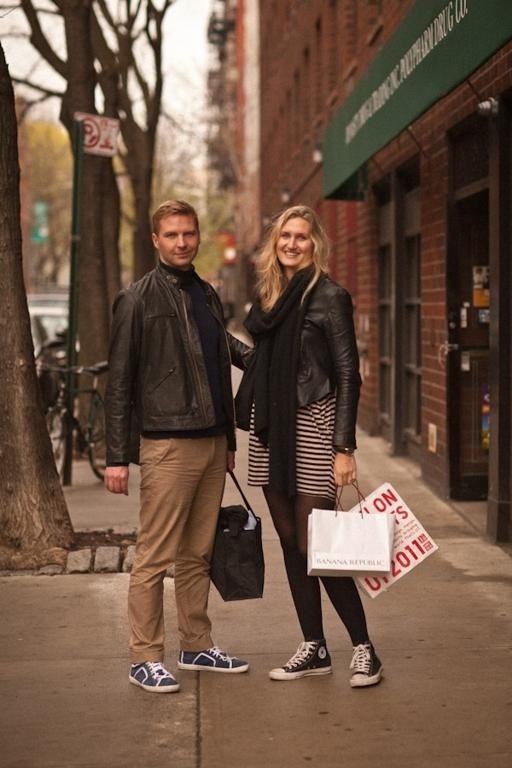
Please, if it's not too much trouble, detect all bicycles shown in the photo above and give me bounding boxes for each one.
[48,360,107,487]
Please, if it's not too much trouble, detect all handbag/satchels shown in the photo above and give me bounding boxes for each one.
[212,462,267,602]
[303,476,442,602]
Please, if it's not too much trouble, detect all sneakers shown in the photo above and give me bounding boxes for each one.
[124,660,182,694]
[346,639,386,690]
[175,641,250,676]
[267,638,335,682]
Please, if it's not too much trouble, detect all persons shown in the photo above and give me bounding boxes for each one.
[233,205,385,688]
[104,199,253,693]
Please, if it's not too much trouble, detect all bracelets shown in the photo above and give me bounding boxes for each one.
[332,446,355,454]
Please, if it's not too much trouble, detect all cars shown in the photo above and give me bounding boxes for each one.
[29,292,70,399]
